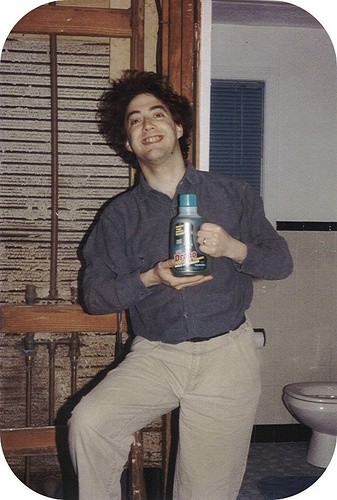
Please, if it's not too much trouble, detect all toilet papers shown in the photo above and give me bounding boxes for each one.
[254,332,264,349]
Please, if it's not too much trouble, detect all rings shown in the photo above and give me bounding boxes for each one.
[202,237,208,245]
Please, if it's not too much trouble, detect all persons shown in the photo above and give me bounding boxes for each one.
[66,66,297,500]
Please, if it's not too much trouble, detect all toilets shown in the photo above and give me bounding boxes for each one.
[282,381,337,468]
